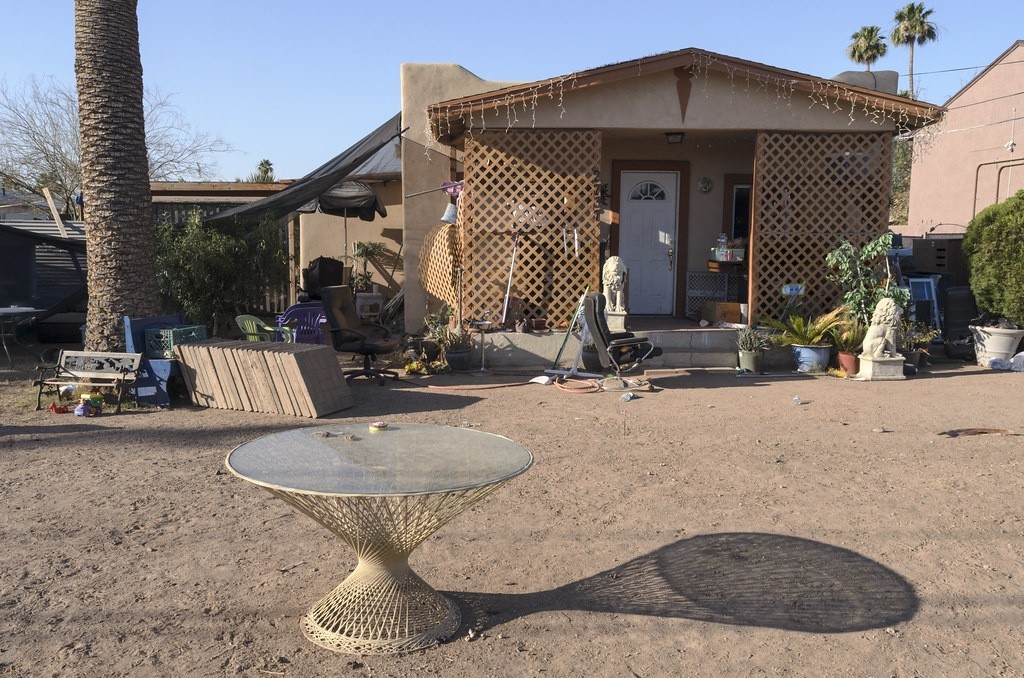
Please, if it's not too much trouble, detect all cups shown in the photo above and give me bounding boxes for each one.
[715,248,734,261]
[373,285,378,294]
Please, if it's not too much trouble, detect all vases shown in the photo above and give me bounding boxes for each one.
[531,318,546,331]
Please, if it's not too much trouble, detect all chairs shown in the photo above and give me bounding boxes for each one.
[321,284,399,386]
[275,302,327,346]
[235,314,291,344]
[584,292,663,392]
[296,257,343,302]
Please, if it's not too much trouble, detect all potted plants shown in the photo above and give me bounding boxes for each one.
[730,302,943,380]
[441,328,471,371]
[961,190,1024,371]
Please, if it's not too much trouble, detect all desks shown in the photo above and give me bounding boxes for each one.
[707,259,747,303]
[0,309,48,369]
[224,425,533,655]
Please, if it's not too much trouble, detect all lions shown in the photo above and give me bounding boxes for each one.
[863,298,904,357]
[601,255,628,312]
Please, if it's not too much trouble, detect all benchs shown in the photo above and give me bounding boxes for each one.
[122,310,185,408]
[32,349,143,414]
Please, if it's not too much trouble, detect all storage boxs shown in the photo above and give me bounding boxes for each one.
[712,246,745,262]
[144,322,207,360]
[701,301,741,325]
[911,238,968,284]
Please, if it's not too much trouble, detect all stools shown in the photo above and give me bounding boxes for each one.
[38,312,87,340]
[354,291,383,325]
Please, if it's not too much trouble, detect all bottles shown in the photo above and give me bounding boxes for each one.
[619,392,633,403]
